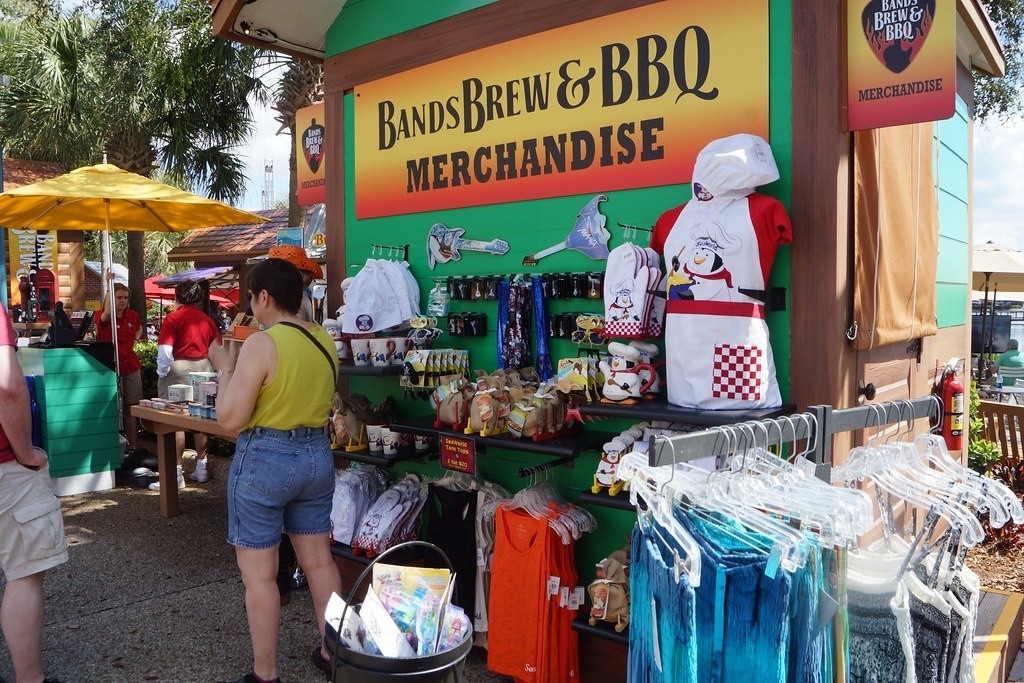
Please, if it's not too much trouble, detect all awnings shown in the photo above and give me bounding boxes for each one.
[245,201,326,268]
[209,288,240,310]
[151,264,236,290]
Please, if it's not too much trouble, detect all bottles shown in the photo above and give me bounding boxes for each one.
[28,281,39,319]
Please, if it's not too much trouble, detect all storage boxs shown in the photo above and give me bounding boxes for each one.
[233,326,258,340]
[167,384,193,401]
[188,372,218,399]
[198,382,216,404]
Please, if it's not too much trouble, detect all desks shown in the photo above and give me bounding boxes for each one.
[981,385,1024,401]
[131,404,237,516]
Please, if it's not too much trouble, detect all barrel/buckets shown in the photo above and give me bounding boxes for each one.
[324,541,473,683]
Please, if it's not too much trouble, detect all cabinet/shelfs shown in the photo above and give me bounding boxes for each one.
[332,288,785,511]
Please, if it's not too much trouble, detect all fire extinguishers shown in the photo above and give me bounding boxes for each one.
[936,363,964,451]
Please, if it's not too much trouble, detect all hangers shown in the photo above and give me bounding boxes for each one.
[420,469,514,502]
[489,464,599,545]
[613,395,1024,588]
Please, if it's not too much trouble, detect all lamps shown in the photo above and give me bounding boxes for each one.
[240,21,251,35]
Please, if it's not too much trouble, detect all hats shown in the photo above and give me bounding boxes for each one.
[345,258,422,333]
[691,133,779,197]
[267,246,323,281]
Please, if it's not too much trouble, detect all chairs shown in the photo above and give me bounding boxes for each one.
[998,366,1024,377]
[977,400,1024,463]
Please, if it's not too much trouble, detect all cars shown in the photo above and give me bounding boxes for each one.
[972,301,1005,305]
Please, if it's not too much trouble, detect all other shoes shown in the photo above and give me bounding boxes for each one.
[216,671,280,683]
[312,647,332,682]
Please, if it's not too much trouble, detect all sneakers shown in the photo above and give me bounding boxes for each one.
[190,466,209,482]
[149,473,185,491]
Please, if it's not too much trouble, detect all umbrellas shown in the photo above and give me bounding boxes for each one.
[144,273,231,334]
[972,239,1024,388]
[0,149,273,449]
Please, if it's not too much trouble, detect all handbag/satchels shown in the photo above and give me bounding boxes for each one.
[336,393,395,446]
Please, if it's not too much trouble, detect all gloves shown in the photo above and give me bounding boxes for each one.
[603,241,668,337]
[352,473,428,554]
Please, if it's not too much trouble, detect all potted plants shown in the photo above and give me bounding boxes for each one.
[133,339,159,400]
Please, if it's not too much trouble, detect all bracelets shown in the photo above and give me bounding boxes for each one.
[133,337,141,344]
[217,368,234,379]
[106,289,110,293]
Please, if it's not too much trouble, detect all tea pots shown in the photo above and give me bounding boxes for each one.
[334,341,351,361]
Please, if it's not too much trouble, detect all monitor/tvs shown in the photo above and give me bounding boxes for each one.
[972,315,1012,353]
[47,308,79,341]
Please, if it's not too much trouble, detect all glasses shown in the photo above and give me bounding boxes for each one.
[247,290,261,301]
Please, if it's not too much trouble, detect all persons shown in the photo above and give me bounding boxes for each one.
[206,256,342,683]
[988,338,1024,400]
[148,278,222,494]
[215,309,233,332]
[92,266,144,459]
[0,298,71,683]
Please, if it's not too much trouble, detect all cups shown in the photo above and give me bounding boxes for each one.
[369,339,396,366]
[366,422,388,451]
[350,339,371,366]
[414,434,433,449]
[188,403,217,420]
[408,338,418,350]
[391,337,409,364]
[381,426,411,454]
[139,398,177,410]
